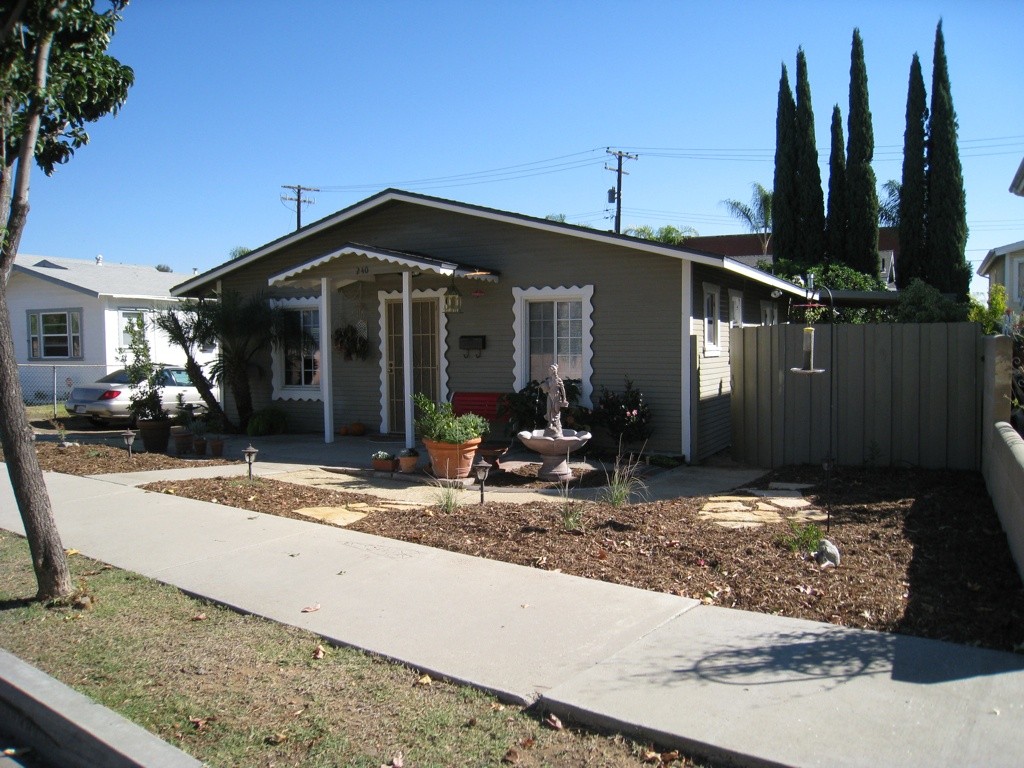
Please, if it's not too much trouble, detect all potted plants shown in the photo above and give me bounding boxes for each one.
[371,451,400,472]
[127,389,172,453]
[411,392,490,480]
[189,418,209,455]
[398,447,420,474]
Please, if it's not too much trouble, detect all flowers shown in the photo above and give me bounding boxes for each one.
[596,374,657,449]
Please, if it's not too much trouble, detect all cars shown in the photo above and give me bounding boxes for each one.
[64,363,220,428]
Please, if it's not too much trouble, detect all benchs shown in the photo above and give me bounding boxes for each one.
[452,392,520,449]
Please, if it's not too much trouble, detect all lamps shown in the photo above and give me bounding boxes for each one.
[120,428,137,456]
[240,443,260,479]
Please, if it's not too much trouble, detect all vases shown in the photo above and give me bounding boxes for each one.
[210,440,224,458]
[339,423,366,437]
[173,430,194,453]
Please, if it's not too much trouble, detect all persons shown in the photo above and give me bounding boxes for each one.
[535,363,568,433]
